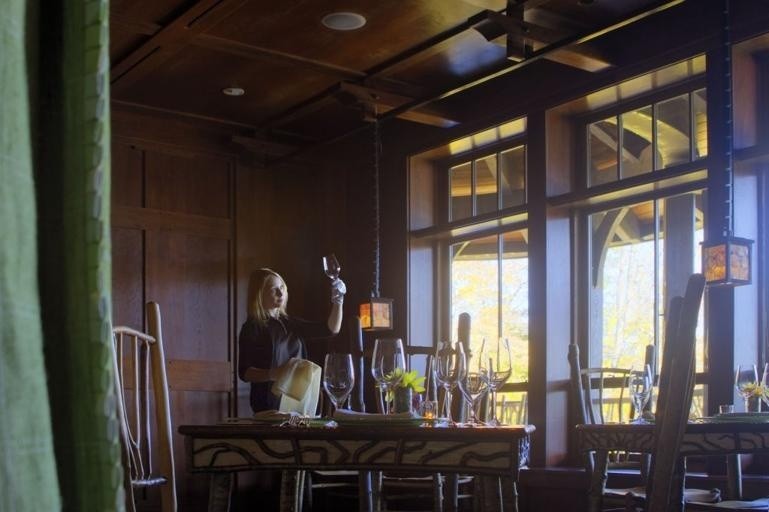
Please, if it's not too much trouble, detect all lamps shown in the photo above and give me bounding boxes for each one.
[356,105,395,333]
[699,0,755,289]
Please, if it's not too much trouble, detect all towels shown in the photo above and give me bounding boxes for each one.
[335,409,416,418]
[271,359,322,418]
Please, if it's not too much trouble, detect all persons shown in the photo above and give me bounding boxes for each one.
[238,267,346,416]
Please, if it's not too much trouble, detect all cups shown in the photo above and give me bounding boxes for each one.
[720,405,734,414]
[433,341,467,427]
[459,351,491,426]
[735,364,759,412]
[628,363,653,425]
[323,255,345,299]
[323,353,355,411]
[478,337,512,426]
[371,338,406,415]
[631,376,648,425]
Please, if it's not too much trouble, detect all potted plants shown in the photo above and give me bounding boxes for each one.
[737,382,769,413]
[375,368,427,413]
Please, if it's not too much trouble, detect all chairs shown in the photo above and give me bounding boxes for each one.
[567,342,722,512]
[111,300,179,512]
[645,272,769,512]
[345,310,473,512]
